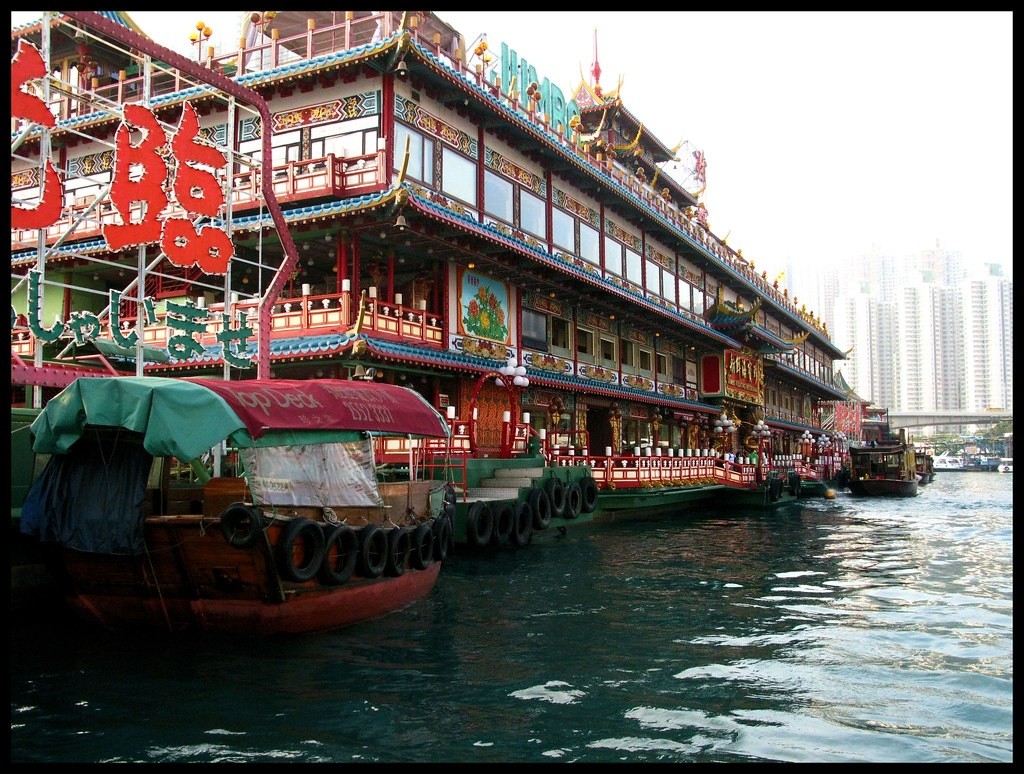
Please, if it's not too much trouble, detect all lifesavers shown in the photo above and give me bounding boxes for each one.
[434,518,449,560]
[544,476,565,518]
[272,518,325,582]
[410,524,434,570]
[581,477,599,513]
[220,504,263,549]
[389,527,410,578]
[564,481,583,519]
[493,503,515,544]
[324,524,358,584]
[358,525,389,578]
[515,501,533,545]
[467,501,493,543]
[529,488,551,530]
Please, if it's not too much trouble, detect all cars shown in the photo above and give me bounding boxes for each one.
[981,457,1000,465]
[963,458,982,467]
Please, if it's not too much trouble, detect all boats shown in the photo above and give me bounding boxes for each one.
[998,458,1013,473]
[31,376,451,643]
[933,451,967,472]
[915,452,937,485]
[848,434,922,498]
[11,10,886,546]
